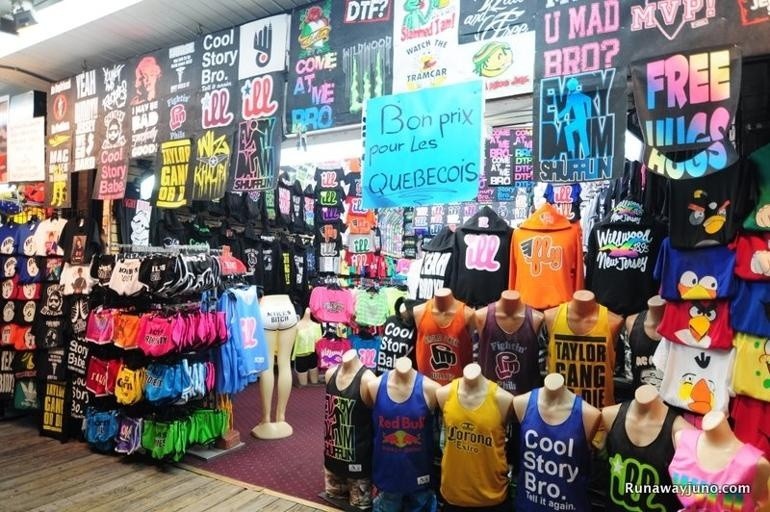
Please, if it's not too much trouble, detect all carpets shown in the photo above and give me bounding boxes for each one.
[173,372,361,510]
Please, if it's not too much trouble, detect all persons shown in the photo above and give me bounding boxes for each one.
[250,294,299,439]
[102,119,124,149]
[45,232,58,256]
[47,291,61,314]
[72,239,85,264]
[291,308,322,385]
[45,331,57,346]
[71,267,87,293]
[131,55,161,105]
[324,287,770,512]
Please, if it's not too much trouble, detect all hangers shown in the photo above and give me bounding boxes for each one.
[80,240,264,426]
[182,212,316,246]
[326,275,408,292]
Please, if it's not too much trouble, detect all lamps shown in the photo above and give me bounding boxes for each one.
[12,0,38,32]
[0,9,19,36]
